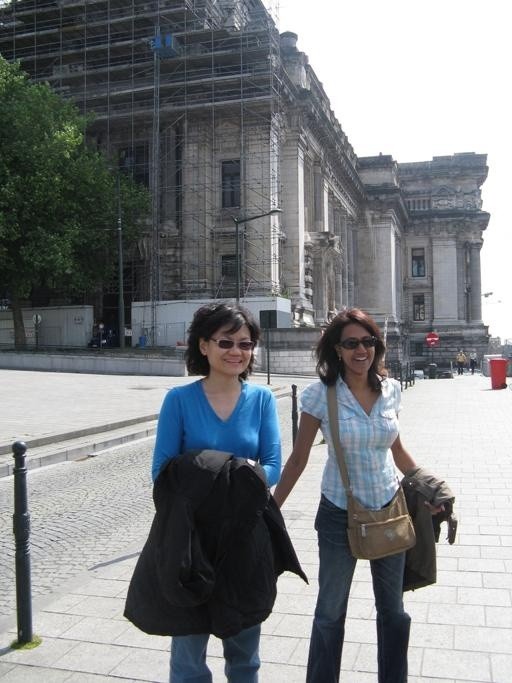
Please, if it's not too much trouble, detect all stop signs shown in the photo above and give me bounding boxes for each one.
[425,333,440,346]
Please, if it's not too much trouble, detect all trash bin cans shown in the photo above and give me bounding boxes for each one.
[488,359,508,390]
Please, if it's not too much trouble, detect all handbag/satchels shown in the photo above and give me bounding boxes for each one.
[347,484,417,560]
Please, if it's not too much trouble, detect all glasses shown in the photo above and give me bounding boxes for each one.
[337,334,378,350]
[208,336,255,350]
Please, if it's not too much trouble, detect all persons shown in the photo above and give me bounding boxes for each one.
[151,301,282,681]
[271,306,447,682]
[469,349,477,374]
[455,350,467,375]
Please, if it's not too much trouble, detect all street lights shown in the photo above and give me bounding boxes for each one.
[230,207,285,303]
[107,166,126,350]
